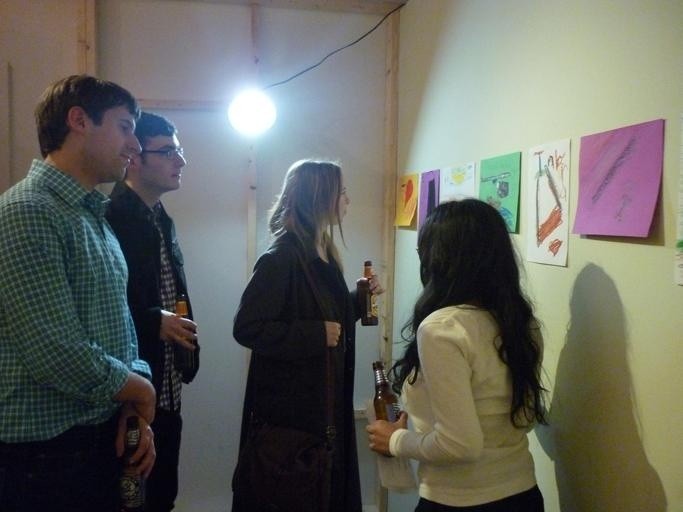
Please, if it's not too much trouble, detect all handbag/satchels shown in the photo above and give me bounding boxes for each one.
[232,424,332,510]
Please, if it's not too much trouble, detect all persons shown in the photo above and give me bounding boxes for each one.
[0,72,157,512]
[105,111,200,512]
[228,158,387,512]
[364,195,553,512]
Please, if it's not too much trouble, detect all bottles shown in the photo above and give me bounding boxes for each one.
[116,414,149,512]
[172,289,196,371]
[355,258,381,326]
[371,359,403,459]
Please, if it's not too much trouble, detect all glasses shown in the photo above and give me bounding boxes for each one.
[142,147,184,161]
